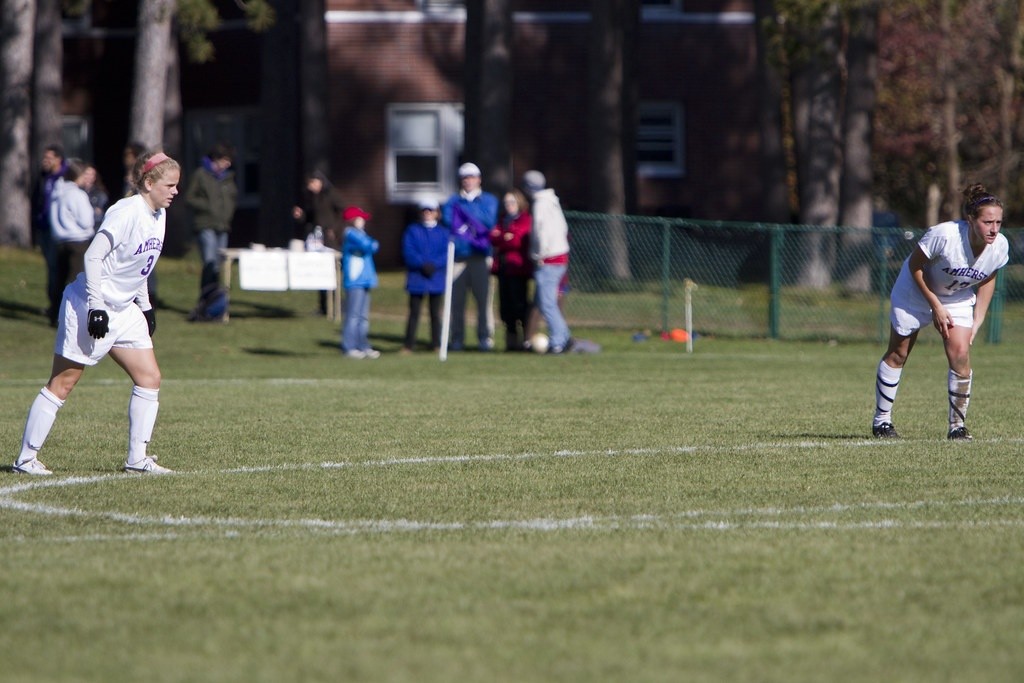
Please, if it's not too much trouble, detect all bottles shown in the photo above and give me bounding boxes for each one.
[313,225,323,252]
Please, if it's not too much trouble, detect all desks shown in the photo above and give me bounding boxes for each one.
[218,245,343,323]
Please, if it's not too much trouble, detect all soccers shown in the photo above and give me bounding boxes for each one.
[531,333,549,353]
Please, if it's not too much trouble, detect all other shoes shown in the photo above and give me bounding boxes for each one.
[433,347,441,356]
[479,338,497,353]
[504,342,531,354]
[450,341,464,352]
[195,307,226,324]
[399,346,412,356]
[542,336,578,358]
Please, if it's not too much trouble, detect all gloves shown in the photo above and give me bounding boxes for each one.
[86,298,110,339]
[420,261,435,279]
[136,295,156,338]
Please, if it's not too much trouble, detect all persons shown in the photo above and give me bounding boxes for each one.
[30,145,107,329]
[185,144,239,320]
[872,183,1009,440]
[11,151,181,475]
[292,171,352,315]
[341,206,381,359]
[125,141,157,304]
[405,163,575,355]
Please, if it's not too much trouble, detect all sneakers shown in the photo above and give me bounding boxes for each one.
[343,348,366,360]
[361,348,380,359]
[872,416,901,440]
[12,458,52,477]
[123,457,173,475]
[947,426,973,442]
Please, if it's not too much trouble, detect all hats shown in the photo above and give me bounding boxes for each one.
[419,195,440,211]
[458,163,480,177]
[521,170,547,193]
[344,207,369,219]
